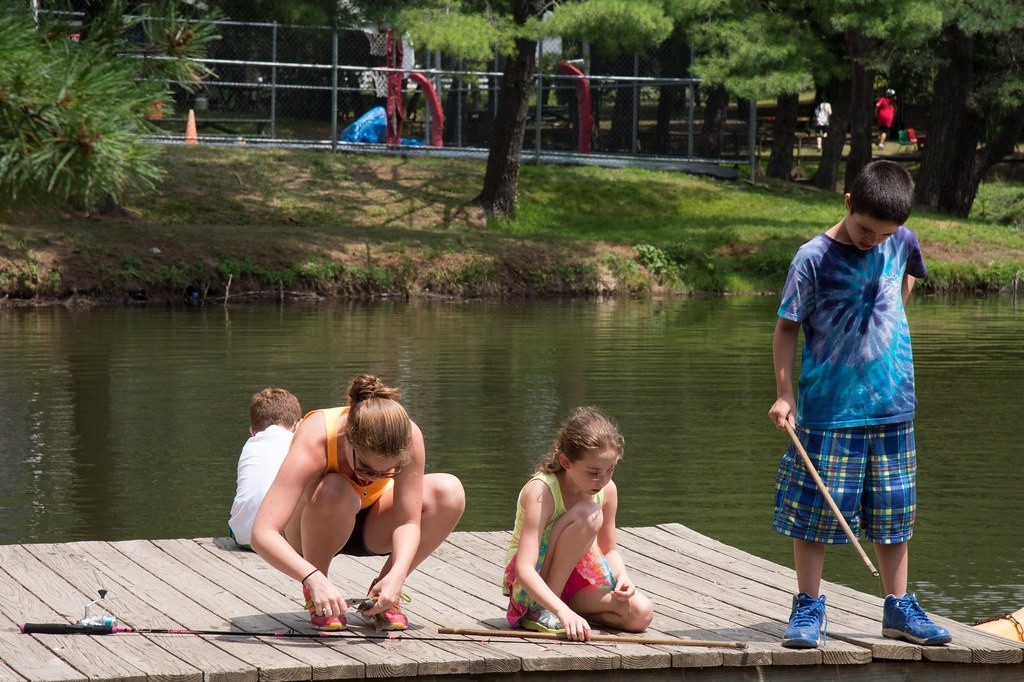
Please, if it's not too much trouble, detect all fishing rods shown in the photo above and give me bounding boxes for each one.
[785,418,881,580]
[17,569,618,647]
[438,625,749,650]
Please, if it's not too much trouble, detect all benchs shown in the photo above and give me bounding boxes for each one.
[150,118,272,135]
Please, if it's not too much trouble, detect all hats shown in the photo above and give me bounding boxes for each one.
[887,89,895,95]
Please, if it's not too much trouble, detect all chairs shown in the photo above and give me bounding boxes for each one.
[895,128,917,155]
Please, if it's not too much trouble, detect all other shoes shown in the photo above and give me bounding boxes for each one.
[878,144,884,149]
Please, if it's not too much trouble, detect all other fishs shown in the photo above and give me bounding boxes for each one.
[358,600,393,633]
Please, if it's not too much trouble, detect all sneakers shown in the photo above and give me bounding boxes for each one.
[782,592,828,647]
[365,578,412,631]
[302,584,348,629]
[522,602,566,633]
[882,591,952,646]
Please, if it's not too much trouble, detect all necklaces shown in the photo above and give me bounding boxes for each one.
[359,478,369,496]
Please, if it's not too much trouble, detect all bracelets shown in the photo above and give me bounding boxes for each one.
[301,569,319,584]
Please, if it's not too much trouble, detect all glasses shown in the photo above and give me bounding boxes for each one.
[352,447,402,479]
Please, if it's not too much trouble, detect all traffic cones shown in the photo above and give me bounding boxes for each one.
[184,109,199,143]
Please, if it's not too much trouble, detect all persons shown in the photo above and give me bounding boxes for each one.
[499,405,654,642]
[875,89,898,147]
[767,162,952,648]
[226,387,303,552]
[812,96,832,151]
[250,374,466,631]
